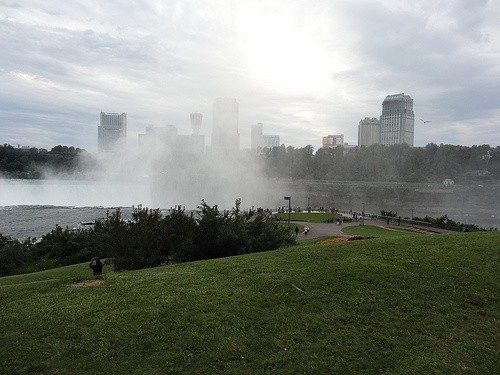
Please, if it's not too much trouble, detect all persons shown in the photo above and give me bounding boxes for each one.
[294,226,299,237]
[304,225,308,237]
[339,217,343,225]
[88,256,105,275]
[398,216,401,225]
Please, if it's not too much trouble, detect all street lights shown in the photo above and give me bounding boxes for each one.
[361,202,365,226]
[283,197,292,229]
[411,209,416,227]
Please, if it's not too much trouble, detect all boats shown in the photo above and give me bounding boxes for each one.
[81,221,96,226]
